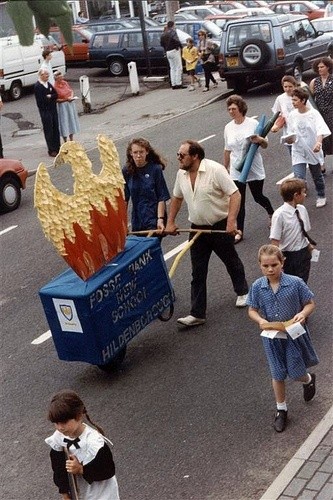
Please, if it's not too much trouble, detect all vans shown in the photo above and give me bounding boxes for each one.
[0,32,66,103]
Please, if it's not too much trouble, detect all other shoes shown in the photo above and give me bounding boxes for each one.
[321,168,326,174]
[50,151,58,157]
[189,85,195,91]
[172,85,177,90]
[202,87,209,92]
[234,233,243,244]
[195,79,202,87]
[213,82,218,88]
[176,84,187,89]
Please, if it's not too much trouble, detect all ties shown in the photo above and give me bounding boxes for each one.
[295,210,317,246]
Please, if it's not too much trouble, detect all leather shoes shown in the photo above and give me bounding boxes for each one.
[274,408,288,433]
[302,372,315,402]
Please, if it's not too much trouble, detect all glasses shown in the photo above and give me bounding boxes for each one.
[177,152,194,159]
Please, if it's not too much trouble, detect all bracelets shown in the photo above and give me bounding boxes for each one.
[158,217,165,219]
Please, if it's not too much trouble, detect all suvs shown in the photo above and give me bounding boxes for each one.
[85,26,197,77]
[218,13,333,95]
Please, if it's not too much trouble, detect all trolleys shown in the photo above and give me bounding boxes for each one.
[39,228,242,376]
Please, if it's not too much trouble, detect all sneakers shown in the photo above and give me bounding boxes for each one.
[316,197,326,208]
[236,293,249,307]
[176,315,206,325]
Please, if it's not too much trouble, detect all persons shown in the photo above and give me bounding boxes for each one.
[223,95,275,244]
[246,245,320,433]
[285,88,331,208]
[309,57,333,174]
[44,391,119,500]
[163,141,250,325]
[40,50,54,85]
[52,71,80,143]
[273,74,311,130]
[197,30,218,92]
[269,177,316,283]
[120,138,172,244]
[160,21,187,89]
[34,69,60,156]
[182,39,203,92]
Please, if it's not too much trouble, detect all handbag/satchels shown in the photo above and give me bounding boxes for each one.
[200,52,212,62]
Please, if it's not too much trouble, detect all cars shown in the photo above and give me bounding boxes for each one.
[0,157,29,214]
[36,0,333,69]
